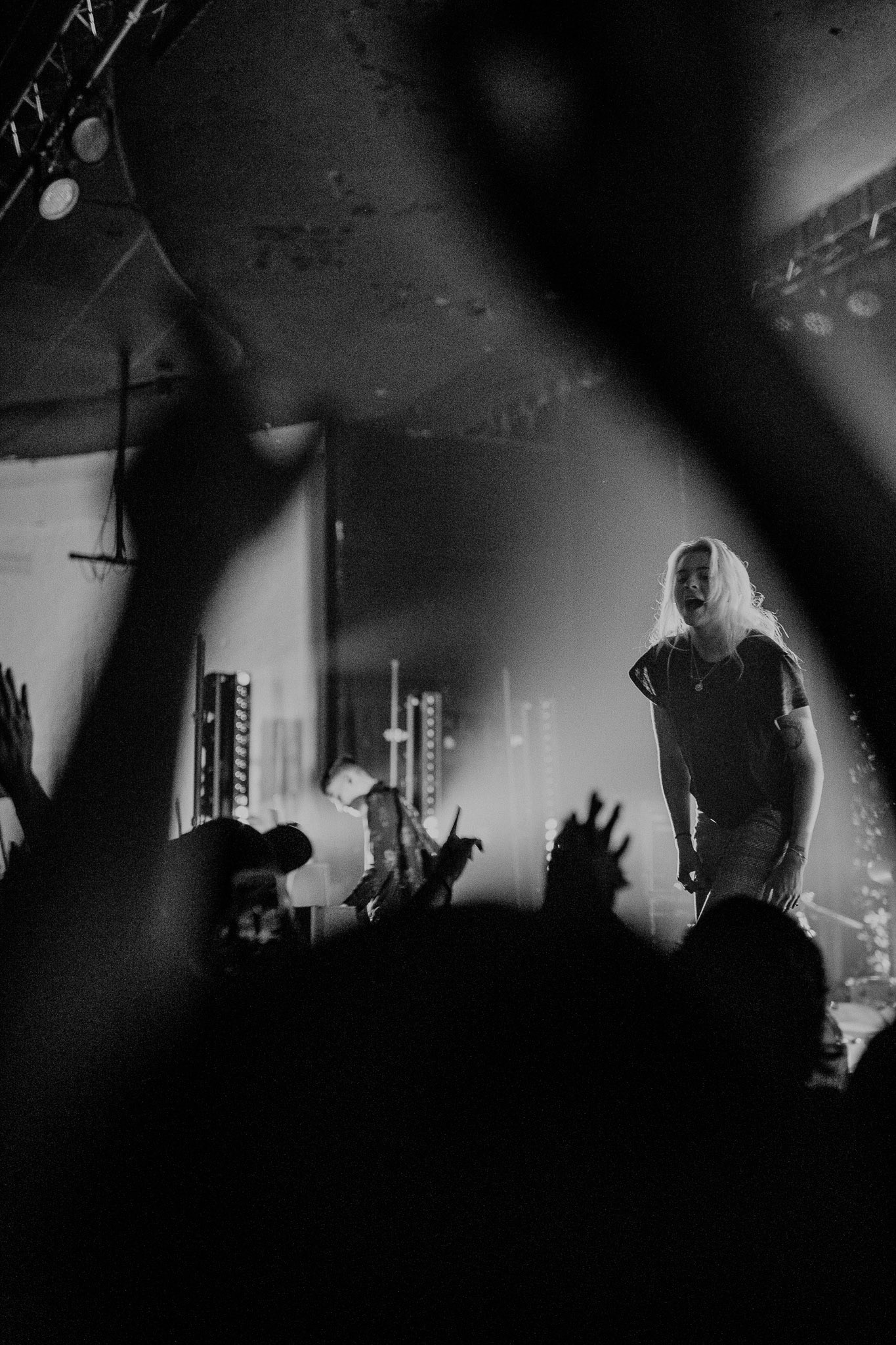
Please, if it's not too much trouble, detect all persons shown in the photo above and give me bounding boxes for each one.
[0,269,896,1345]
[627,535,825,926]
[320,755,438,925]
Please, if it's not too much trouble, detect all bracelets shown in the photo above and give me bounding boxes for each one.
[783,844,809,865]
[674,831,692,839]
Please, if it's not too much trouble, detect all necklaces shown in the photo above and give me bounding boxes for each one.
[684,624,750,692]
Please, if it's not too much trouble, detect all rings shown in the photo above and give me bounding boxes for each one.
[779,905,786,910]
[787,906,793,909]
[795,902,800,906]
[683,883,689,891]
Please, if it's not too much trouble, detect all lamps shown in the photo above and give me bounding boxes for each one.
[33,99,110,220]
